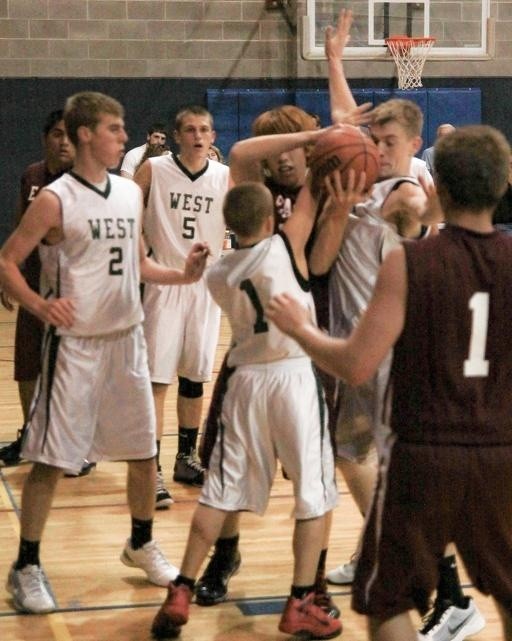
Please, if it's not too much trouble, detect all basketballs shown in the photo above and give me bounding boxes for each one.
[309,124,382,192]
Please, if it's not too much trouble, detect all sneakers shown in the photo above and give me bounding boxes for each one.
[121,537,240,638]
[0,428,25,466]
[279,558,358,639]
[6,561,56,614]
[417,596,485,641]
[155,453,206,508]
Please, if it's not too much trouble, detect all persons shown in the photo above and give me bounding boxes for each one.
[263,126,509,640]
[3,7,512,639]
[1,93,213,616]
